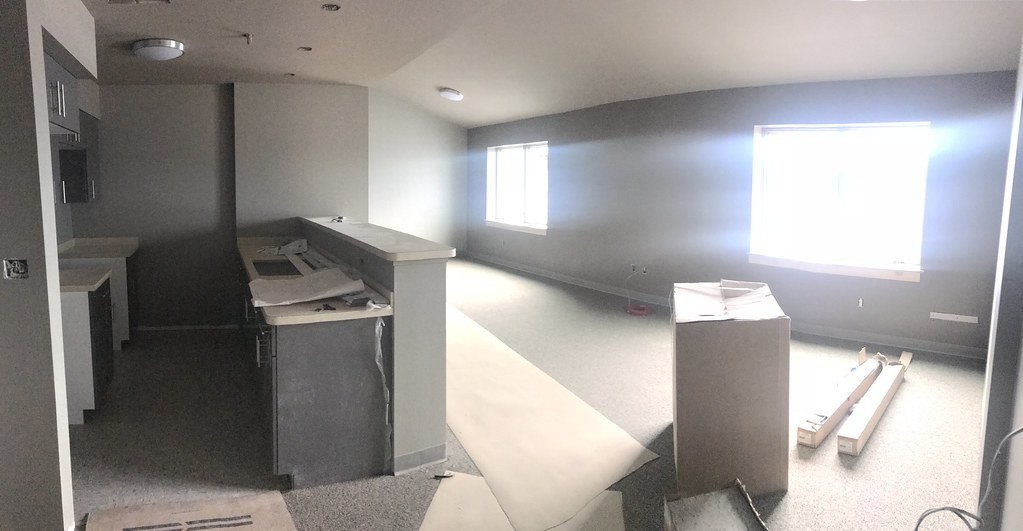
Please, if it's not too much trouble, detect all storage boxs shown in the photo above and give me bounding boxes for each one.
[668,278,914,500]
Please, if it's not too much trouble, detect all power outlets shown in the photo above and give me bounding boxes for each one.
[631,263,637,273]
[640,266,649,275]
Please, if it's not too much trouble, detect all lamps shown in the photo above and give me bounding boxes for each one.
[131,38,187,62]
[439,86,463,99]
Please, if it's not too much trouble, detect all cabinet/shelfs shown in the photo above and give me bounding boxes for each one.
[36,43,392,491]
